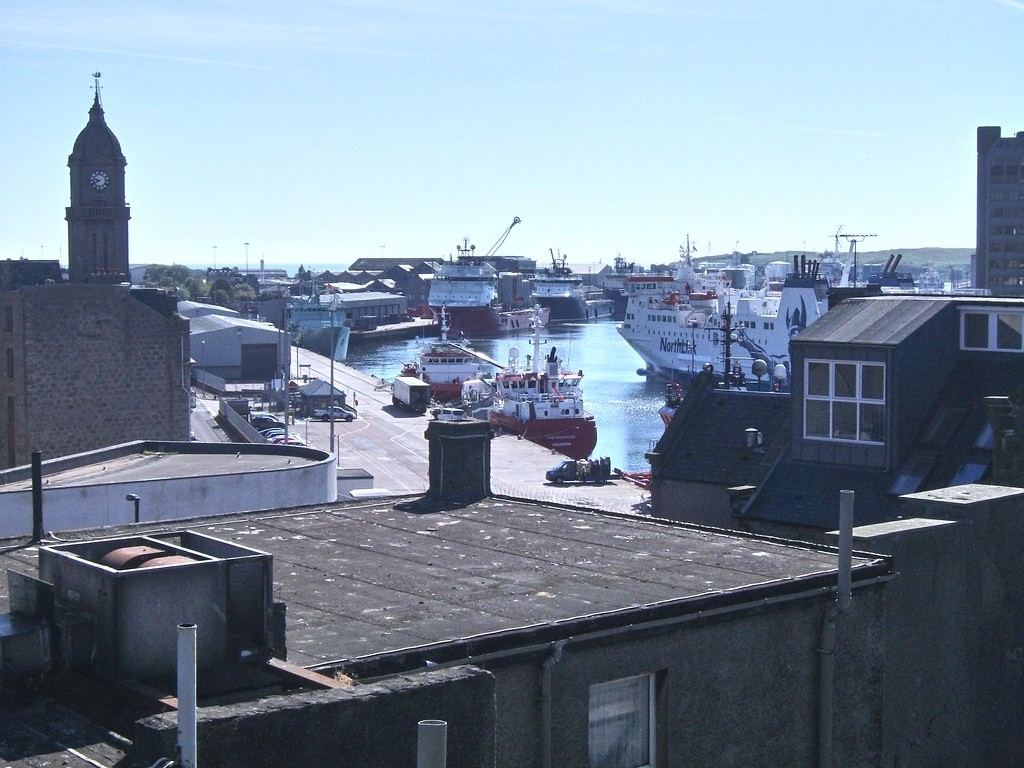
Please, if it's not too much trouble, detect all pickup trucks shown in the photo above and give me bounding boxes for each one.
[313,405,356,422]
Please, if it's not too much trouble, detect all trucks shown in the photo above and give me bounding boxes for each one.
[391,377,431,414]
[546,460,611,485]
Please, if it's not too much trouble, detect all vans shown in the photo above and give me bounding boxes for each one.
[432,408,468,422]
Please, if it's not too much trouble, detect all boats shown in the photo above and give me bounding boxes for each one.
[602,232,904,429]
[427,234,553,342]
[456,300,598,462]
[523,249,617,325]
[398,300,481,407]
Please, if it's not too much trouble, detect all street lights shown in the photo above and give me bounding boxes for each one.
[243,243,250,286]
[213,246,219,304]
[125,492,141,524]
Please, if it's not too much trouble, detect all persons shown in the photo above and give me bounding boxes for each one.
[581,466,587,484]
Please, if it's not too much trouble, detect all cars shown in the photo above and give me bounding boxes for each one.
[248,410,312,447]
